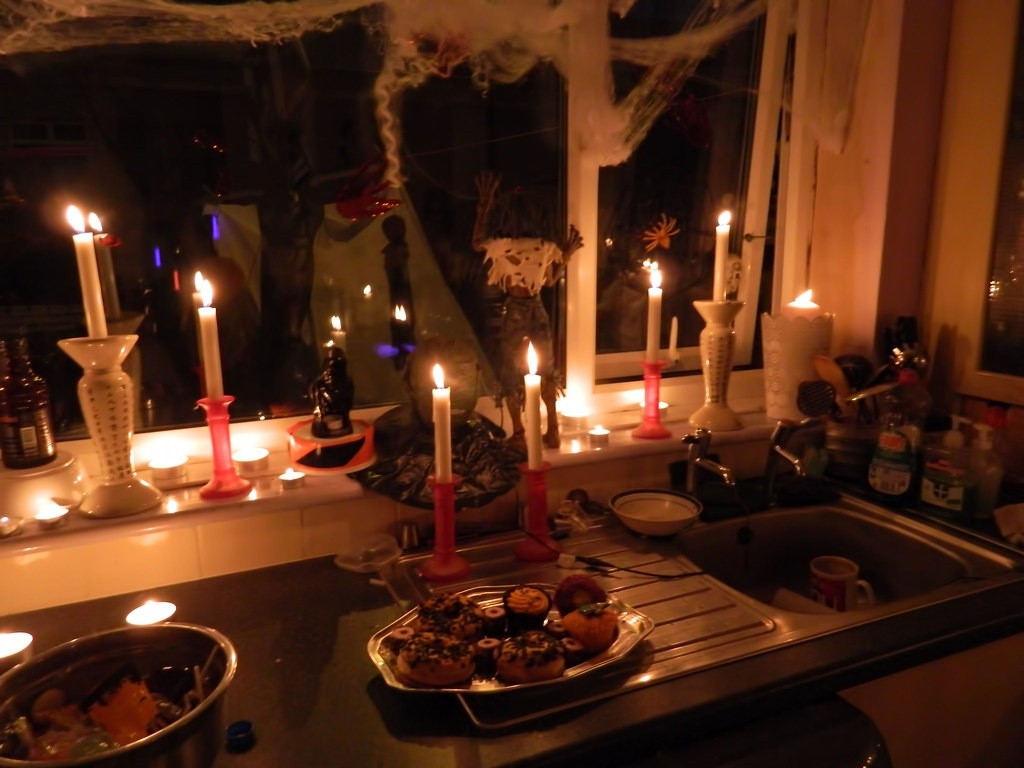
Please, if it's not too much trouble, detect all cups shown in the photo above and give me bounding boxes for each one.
[823,416,882,490]
[809,555,874,612]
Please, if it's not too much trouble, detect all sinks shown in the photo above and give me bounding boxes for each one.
[677,481,1024,645]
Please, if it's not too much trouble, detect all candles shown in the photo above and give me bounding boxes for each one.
[65,204,108,338]
[149,449,188,480]
[198,279,225,401]
[331,314,347,359]
[639,400,669,418]
[0,516,24,538]
[713,210,732,302]
[35,495,69,531]
[191,271,205,372]
[278,467,306,489]
[125,601,176,625]
[588,425,610,445]
[431,363,453,484]
[0,632,34,672]
[523,341,543,471]
[784,288,821,321]
[88,211,122,323]
[231,447,270,473]
[646,269,664,365]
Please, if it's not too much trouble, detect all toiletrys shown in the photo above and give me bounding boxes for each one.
[856,385,1003,523]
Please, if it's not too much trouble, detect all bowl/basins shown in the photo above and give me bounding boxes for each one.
[608,488,703,536]
[0,622,238,768]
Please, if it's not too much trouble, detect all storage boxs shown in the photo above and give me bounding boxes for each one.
[921,478,972,511]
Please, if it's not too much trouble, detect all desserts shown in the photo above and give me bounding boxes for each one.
[495,634,565,680]
[563,608,616,650]
[561,638,583,656]
[503,585,553,632]
[477,638,499,674]
[556,575,607,616]
[416,592,482,637]
[483,607,505,632]
[397,632,475,685]
[390,626,415,652]
[548,621,568,639]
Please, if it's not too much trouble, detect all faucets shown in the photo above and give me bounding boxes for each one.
[763,414,824,501]
[674,427,736,498]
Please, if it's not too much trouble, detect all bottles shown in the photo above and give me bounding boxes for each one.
[881,368,932,450]
[0,337,58,470]
